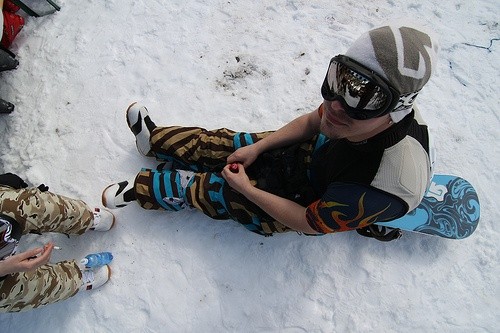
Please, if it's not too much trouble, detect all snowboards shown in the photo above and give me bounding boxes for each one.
[160,161,480,240]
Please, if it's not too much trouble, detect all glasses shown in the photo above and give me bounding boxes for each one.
[320,54,400,121]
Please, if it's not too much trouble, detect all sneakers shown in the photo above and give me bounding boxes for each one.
[88,207,115,232]
[126,102,158,159]
[78,264,111,292]
[101,174,139,209]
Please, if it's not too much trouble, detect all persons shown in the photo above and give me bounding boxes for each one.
[0,185,115,314]
[102,25,436,236]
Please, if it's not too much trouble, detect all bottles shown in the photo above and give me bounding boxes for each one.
[80,252,112,267]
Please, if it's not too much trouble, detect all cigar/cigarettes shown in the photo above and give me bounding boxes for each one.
[45,245,62,250]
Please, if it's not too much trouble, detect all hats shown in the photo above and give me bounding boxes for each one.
[344,23,440,122]
